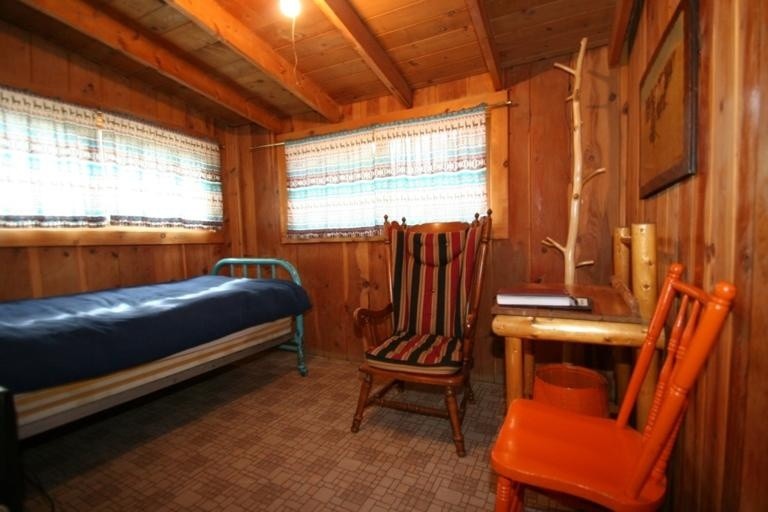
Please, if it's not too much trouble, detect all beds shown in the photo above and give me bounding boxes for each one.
[0,258,312,512]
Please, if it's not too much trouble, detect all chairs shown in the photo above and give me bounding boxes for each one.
[490,263,737,512]
[350,208,493,455]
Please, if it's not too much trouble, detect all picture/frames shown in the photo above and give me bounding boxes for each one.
[638,0,694,200]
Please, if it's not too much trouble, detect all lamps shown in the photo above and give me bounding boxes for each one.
[279,0,302,87]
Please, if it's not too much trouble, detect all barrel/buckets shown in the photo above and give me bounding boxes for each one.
[533,364,608,417]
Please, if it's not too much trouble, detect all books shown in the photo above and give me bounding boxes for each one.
[496,287,593,312]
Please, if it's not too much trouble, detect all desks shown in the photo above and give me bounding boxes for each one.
[491,225,655,494]
